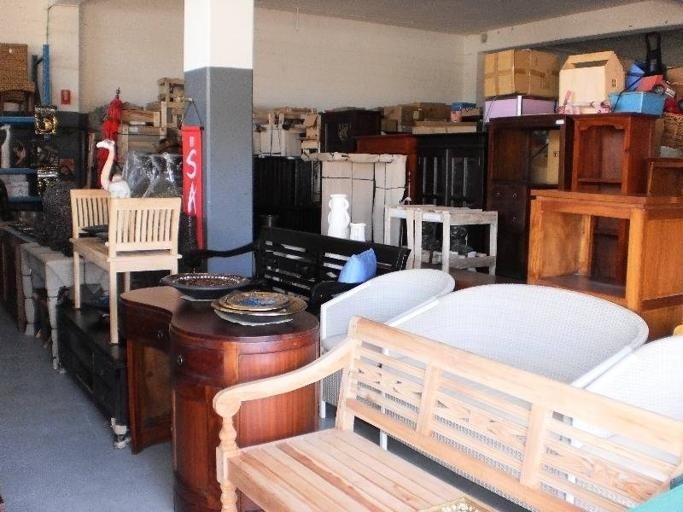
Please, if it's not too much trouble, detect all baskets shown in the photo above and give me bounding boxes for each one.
[663,112,683,148]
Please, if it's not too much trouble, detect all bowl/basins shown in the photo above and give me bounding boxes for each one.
[159,272,251,297]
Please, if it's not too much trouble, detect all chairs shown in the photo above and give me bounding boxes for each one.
[0,42,36,114]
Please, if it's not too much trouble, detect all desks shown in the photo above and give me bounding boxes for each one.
[0,220,109,371]
[117,284,319,511]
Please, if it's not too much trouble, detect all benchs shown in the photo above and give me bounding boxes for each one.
[186,224,410,327]
[68,188,182,344]
[210,316,680,512]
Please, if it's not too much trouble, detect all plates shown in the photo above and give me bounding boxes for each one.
[213,291,306,327]
[82,223,108,239]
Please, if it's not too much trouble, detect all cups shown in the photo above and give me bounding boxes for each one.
[450,110,462,124]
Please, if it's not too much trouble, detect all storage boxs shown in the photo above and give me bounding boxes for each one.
[608,90,666,117]
[380,100,482,136]
[484,50,556,100]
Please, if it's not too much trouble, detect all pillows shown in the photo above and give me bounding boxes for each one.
[333,246,376,296]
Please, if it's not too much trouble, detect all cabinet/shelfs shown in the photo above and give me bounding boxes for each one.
[353,133,416,204]
[0,117,49,202]
[56,305,129,446]
[418,131,486,243]
[383,204,499,279]
[318,111,382,154]
